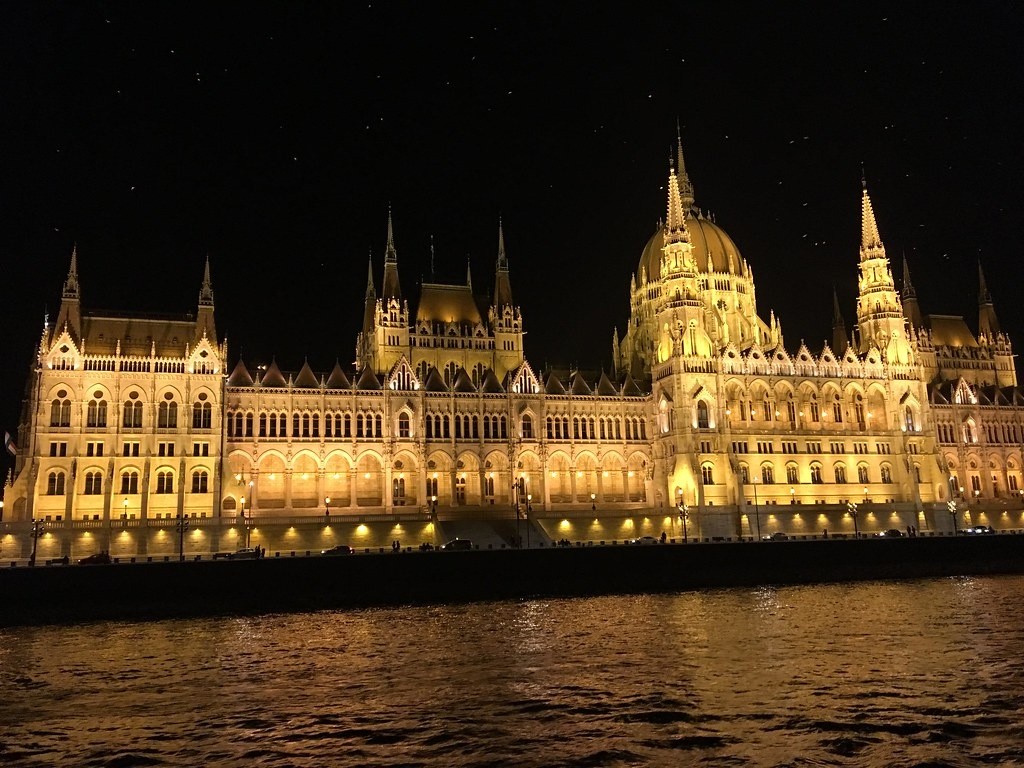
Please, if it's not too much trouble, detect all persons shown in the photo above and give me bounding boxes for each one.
[907,525,915,535]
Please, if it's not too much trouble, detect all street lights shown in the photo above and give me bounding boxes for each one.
[431,496,435,550]
[679,488,688,544]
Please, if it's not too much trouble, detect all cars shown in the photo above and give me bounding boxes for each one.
[963,525,989,534]
[78,553,112,565]
[762,533,789,541]
[228,547,255,558]
[439,538,473,551]
[871,529,905,537]
[320,545,355,555]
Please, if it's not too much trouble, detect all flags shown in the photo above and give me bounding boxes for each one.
[5,432,17,456]
